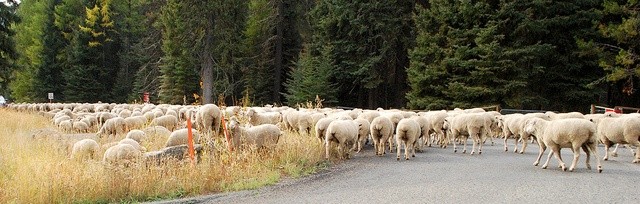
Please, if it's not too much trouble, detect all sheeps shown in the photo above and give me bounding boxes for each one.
[4,102,640,173]
